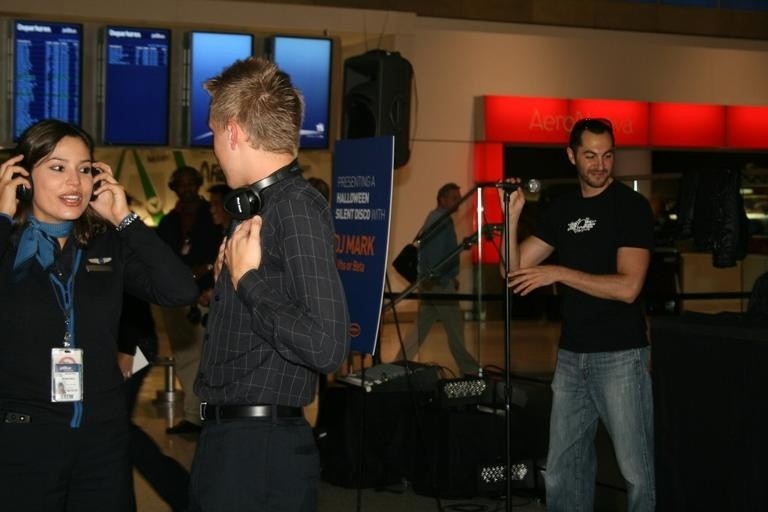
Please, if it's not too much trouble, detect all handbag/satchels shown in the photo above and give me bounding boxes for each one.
[393,245,417,284]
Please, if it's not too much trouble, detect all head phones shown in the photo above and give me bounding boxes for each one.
[8,117,102,205]
[220,157,303,219]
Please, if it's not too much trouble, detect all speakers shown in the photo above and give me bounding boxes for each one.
[339,50,414,170]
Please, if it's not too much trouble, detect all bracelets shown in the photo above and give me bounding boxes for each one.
[117,214,137,232]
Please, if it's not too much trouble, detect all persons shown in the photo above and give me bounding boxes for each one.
[118,252,189,511]
[497,118,659,511]
[187,57,352,511]
[0,116,196,512]
[154,165,220,304]
[200,180,239,311]
[392,183,482,380]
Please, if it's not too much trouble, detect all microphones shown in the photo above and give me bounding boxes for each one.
[470,178,545,194]
[488,222,507,234]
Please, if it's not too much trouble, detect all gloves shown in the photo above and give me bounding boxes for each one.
[199,403,303,421]
